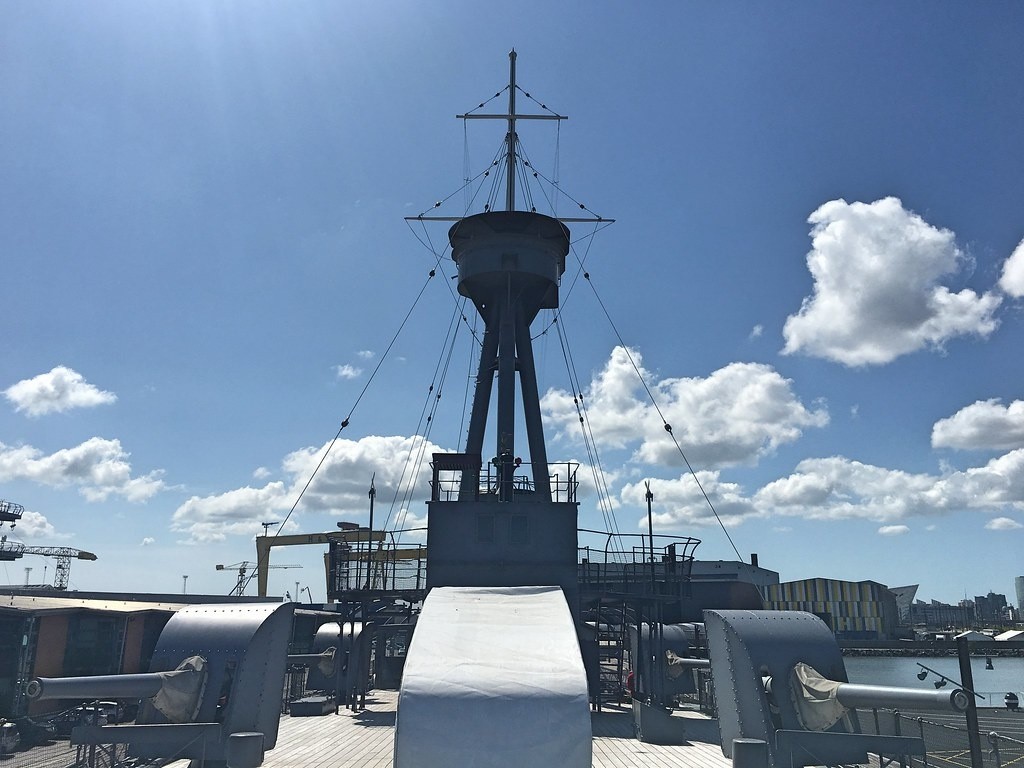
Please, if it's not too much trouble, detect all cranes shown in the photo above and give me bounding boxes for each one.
[216,561,305,596]
